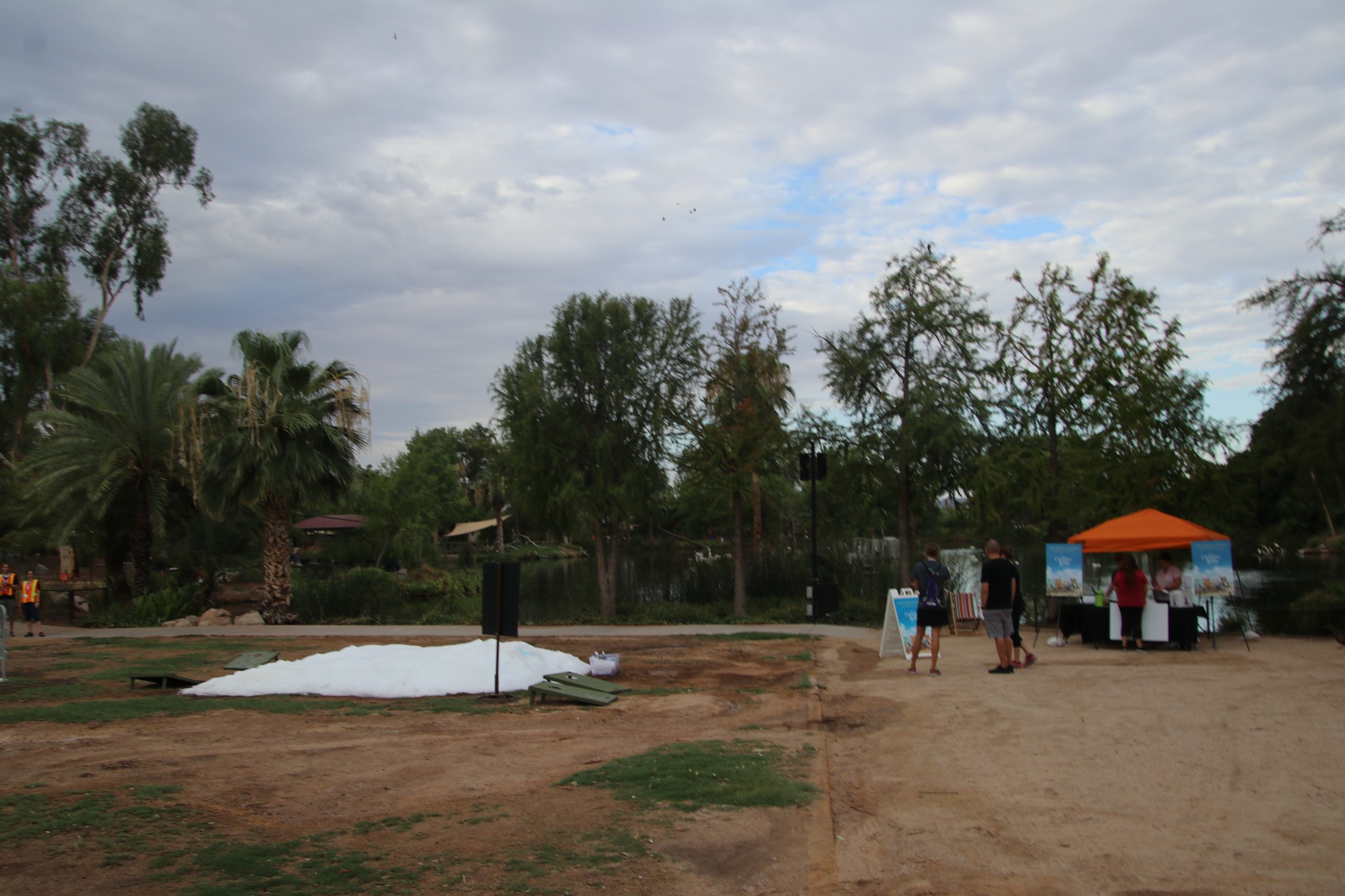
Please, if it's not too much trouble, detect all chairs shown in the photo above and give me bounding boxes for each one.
[949,592,983,636]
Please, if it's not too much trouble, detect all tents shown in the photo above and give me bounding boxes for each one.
[1056,508,1252,653]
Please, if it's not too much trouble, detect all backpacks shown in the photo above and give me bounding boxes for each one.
[916,561,946,615]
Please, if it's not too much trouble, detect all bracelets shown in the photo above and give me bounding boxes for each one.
[981,607,986,610]
[36,601,40,603]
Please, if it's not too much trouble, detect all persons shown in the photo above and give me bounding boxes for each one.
[18,570,45,637]
[1153,553,1181,592]
[1002,546,1038,668]
[908,544,950,675]
[1111,553,1125,582]
[1113,554,1149,651]
[981,539,1013,674]
[0,564,19,637]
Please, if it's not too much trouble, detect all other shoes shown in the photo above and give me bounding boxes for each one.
[929,667,942,676]
[1012,660,1023,668]
[10,631,15,637]
[908,665,917,674]
[988,665,1014,674]
[1121,647,1128,653]
[1136,648,1143,652]
[39,632,45,637]
[1023,653,1037,668]
[24,632,33,637]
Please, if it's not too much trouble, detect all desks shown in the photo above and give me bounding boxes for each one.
[1055,604,1207,652]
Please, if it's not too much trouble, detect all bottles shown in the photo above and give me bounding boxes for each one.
[1094,589,1105,607]
